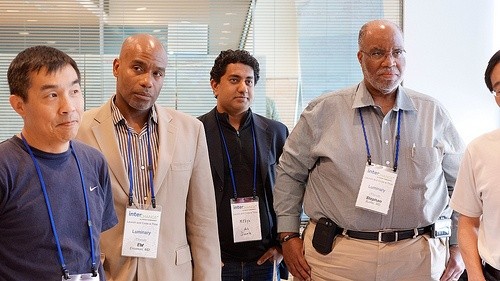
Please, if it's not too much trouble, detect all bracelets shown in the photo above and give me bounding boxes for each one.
[280,233,301,243]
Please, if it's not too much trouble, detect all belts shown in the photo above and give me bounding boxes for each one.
[483,263,500,281]
[341,224,434,243]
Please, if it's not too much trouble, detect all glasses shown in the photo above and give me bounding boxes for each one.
[492,89,500,98]
[360,49,406,58]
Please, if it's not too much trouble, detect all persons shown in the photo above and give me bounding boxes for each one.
[273,19,467,281]
[73,34,222,281]
[196,50,289,281]
[449,51,500,281]
[0,45,119,281]
